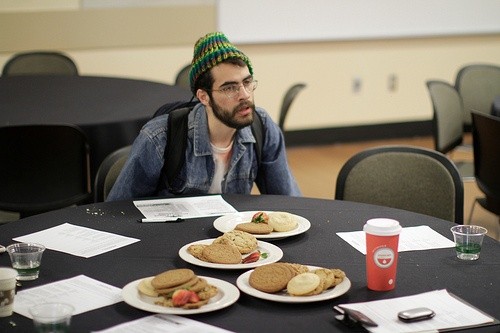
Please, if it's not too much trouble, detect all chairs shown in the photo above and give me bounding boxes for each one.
[0,51,500,223]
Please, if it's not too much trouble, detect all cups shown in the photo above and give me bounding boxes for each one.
[451,225,489,261]
[27,302,76,333]
[362,216,403,293]
[5,242,45,281]
[0,268,19,317]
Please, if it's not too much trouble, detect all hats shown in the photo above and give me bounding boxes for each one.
[189,32,254,96]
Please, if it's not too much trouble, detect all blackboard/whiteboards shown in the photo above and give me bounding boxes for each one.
[218,0,499,45]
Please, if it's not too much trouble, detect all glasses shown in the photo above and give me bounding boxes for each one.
[208,80,259,96]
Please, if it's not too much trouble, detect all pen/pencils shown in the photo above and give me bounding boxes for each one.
[136,217,184,223]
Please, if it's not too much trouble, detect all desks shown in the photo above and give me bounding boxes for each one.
[0,194,500,333]
[0,73,197,206]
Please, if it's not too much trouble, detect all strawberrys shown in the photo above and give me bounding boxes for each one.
[252,212,269,224]
[187,291,199,303]
[242,252,261,263]
[172,287,191,305]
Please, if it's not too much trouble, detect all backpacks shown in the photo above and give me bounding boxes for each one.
[133,100,265,196]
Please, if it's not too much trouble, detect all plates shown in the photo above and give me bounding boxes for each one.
[236,265,351,302]
[213,211,311,239]
[121,275,240,314]
[179,238,285,270]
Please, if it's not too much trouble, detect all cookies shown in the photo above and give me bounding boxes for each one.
[138,268,218,309]
[249,263,345,296]
[234,212,299,233]
[188,229,258,264]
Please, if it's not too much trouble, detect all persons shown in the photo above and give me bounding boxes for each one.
[104,32,302,204]
